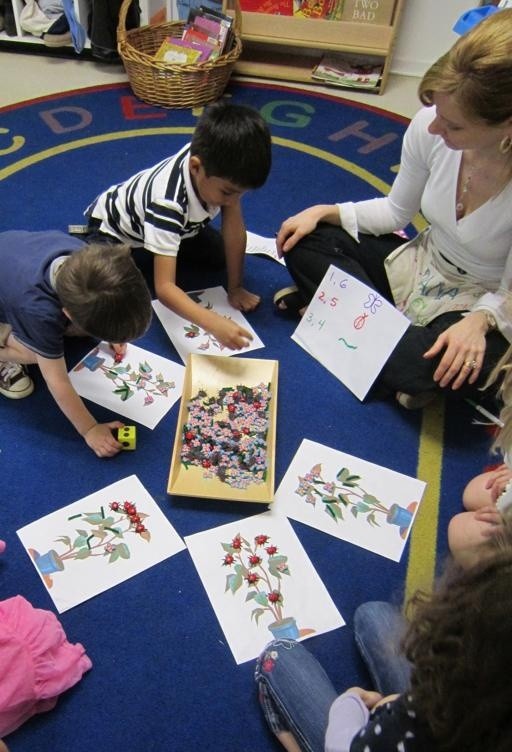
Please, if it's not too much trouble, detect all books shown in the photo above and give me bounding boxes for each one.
[324,80,354,88]
[310,52,383,88]
[132,4,236,90]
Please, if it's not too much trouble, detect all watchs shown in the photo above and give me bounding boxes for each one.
[478,309,497,330]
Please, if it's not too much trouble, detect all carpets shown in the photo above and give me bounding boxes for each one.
[0,78,511,752]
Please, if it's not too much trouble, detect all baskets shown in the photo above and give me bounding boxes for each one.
[116,0,243,110]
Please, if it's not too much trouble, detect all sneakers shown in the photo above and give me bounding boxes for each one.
[0,361,34,399]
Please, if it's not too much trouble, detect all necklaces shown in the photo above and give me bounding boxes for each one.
[455,149,497,221]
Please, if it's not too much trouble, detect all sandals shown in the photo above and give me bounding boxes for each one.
[274,287,306,316]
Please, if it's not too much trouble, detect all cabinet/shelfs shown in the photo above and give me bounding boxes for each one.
[2,1,181,60]
[216,0,403,95]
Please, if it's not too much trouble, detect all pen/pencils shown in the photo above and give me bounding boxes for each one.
[460,391,507,430]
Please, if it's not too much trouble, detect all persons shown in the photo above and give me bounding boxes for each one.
[0,229,153,459]
[254,549,512,751]
[447,365,512,568]
[84,101,272,351]
[0,594,94,752]
[273,6,512,411]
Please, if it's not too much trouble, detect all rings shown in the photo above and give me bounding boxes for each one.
[463,360,481,369]
